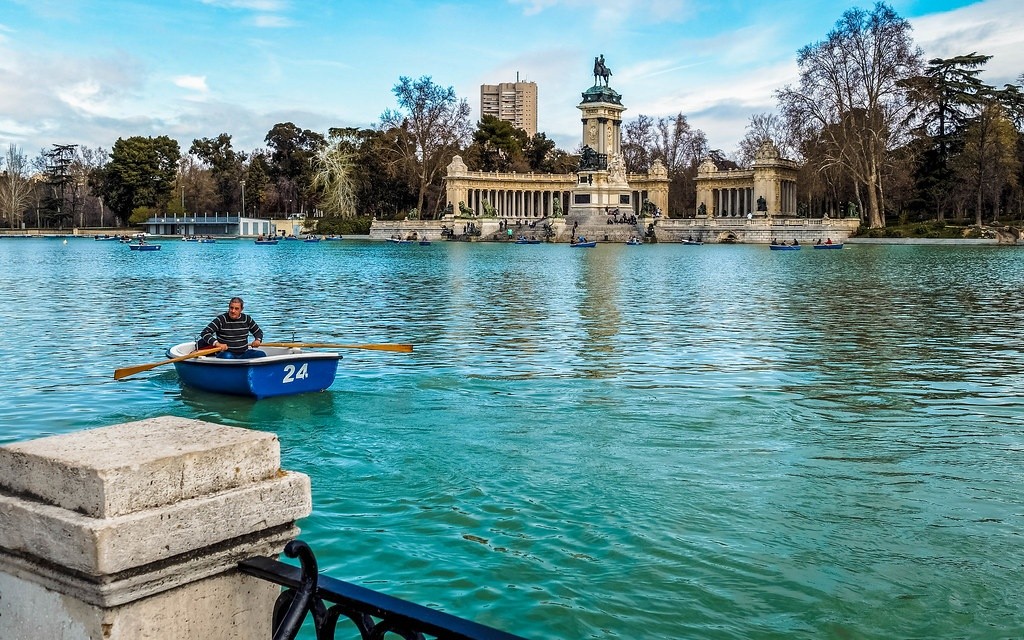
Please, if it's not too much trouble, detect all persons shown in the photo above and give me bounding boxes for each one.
[258,235,277,241]
[824,238,832,244]
[817,239,821,245]
[688,235,693,241]
[201,297,266,359]
[398,233,427,242]
[652,207,662,219]
[499,218,536,240]
[605,204,637,224]
[628,236,636,242]
[576,237,587,243]
[139,239,144,246]
[310,234,342,240]
[757,196,766,211]
[95,233,129,240]
[594,54,605,76]
[747,212,752,220]
[772,238,798,246]
[183,234,213,239]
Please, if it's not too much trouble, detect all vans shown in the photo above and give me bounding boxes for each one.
[287,213,306,220]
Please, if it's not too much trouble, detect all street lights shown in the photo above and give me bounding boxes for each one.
[180,186,185,208]
[239,180,246,218]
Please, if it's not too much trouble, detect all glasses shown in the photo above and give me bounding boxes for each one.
[228,306,240,311]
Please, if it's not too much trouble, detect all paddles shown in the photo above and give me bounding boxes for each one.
[114,346,222,380]
[248,343,413,353]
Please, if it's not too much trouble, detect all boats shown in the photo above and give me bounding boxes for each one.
[769,245,802,250]
[626,241,642,245]
[254,240,279,245]
[326,237,343,241]
[515,240,541,244]
[165,340,345,398]
[385,238,414,244]
[266,236,284,240]
[181,237,216,243]
[419,241,431,245]
[120,238,132,243]
[285,237,299,240]
[94,235,121,241]
[813,243,844,249]
[129,244,162,250]
[570,241,597,247]
[303,237,322,242]
[682,239,704,245]
